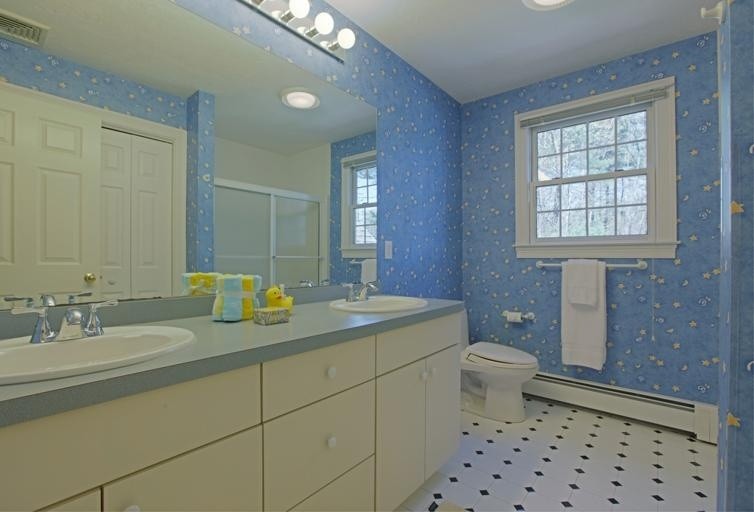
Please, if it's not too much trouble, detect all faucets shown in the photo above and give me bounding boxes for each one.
[56,309,86,341]
[299,278,314,287]
[357,281,378,300]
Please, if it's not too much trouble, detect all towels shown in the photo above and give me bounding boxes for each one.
[359,258,378,285]
[561,258,606,371]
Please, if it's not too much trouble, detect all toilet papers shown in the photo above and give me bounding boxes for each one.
[505,311,524,325]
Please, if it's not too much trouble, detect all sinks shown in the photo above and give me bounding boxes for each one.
[0,323,196,385]
[330,294,429,311]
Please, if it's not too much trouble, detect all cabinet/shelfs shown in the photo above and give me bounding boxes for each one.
[0,79,186,305]
[0,310,461,512]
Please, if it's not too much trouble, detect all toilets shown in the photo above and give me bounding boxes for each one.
[461,310,539,423]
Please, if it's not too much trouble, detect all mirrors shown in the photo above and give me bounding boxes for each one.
[0,1,377,311]
